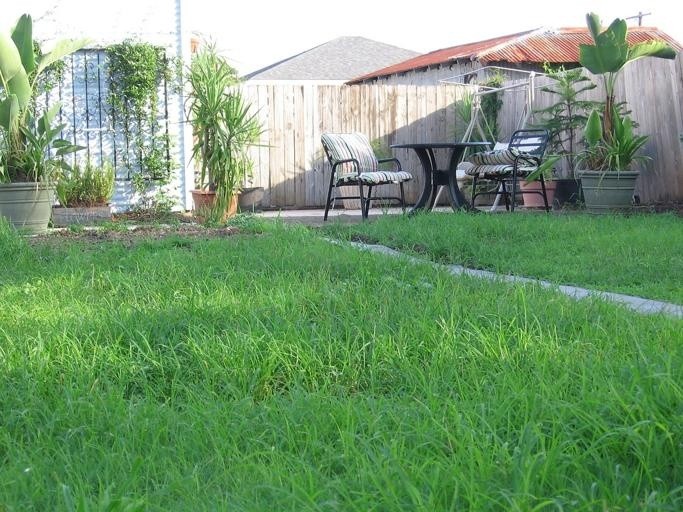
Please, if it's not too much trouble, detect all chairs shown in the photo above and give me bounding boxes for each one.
[319,132,413,226]
[465,129,550,212]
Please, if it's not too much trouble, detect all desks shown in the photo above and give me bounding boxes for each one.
[388,141,496,219]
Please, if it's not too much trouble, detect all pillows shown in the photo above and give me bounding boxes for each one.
[468,147,532,164]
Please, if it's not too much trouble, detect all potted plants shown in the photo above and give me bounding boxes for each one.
[174,32,265,228]
[0,13,117,238]
[518,11,678,215]
